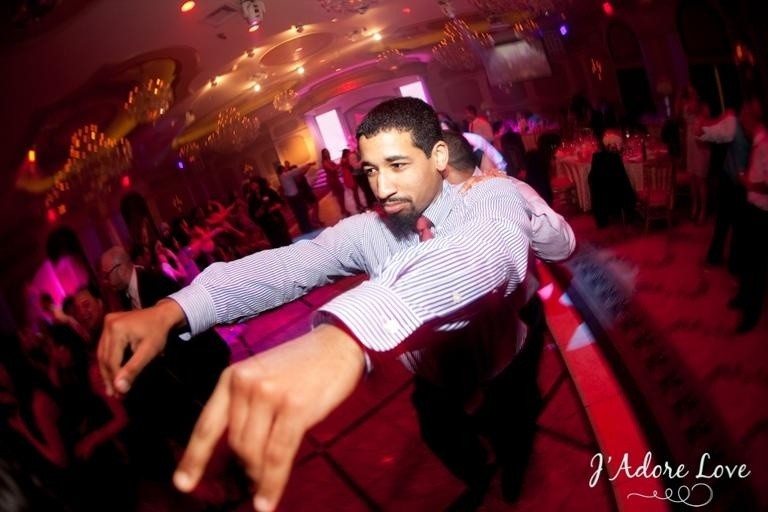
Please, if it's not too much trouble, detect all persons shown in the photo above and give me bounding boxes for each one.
[91,95,531,512]
[726,94,767,341]
[431,128,582,447]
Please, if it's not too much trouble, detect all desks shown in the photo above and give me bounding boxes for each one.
[492,118,673,213]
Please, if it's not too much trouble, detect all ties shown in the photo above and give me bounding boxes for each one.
[413,215,435,244]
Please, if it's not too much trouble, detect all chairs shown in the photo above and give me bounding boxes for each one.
[633,157,675,235]
